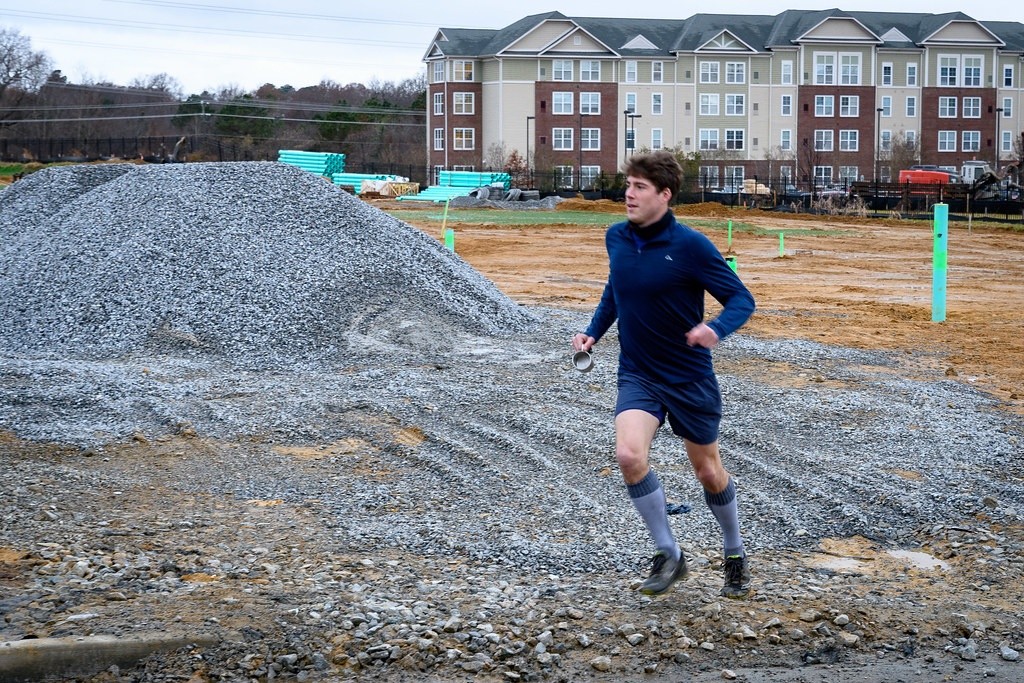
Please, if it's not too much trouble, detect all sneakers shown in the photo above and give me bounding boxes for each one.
[721,548,750,598]
[639,550,687,594]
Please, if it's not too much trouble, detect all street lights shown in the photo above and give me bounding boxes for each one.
[526,116,535,169]
[996,107,1004,168]
[623,110,631,158]
[628,114,642,155]
[877,108,884,165]
[580,114,590,189]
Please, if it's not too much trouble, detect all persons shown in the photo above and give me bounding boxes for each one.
[572,144,757,595]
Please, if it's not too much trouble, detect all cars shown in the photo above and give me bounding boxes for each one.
[784,184,798,191]
[718,187,738,193]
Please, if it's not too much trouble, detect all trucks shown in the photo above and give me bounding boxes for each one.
[899,160,991,195]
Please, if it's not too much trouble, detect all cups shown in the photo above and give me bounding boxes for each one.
[571,345,594,373]
